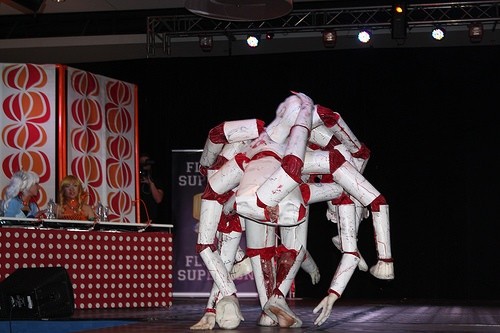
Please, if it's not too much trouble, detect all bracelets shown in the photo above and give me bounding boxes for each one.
[88,216,95,221]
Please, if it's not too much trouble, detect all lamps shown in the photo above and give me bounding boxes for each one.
[198,21,484,52]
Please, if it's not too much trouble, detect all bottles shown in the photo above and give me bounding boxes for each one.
[96,200,102,221]
[104,204,109,221]
[46,198,56,219]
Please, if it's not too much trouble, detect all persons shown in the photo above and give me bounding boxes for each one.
[188,90,396,329]
[1,171,48,219]
[139,157,164,222]
[56,174,96,221]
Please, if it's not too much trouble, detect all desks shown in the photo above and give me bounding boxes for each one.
[0,217,173,311]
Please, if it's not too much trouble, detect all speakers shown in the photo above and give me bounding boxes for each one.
[0,267,74,321]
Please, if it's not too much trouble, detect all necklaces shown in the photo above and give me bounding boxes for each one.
[19,195,29,206]
[65,200,80,209]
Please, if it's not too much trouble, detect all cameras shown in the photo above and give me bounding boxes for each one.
[140,168,148,178]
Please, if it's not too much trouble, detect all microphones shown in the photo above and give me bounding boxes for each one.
[132,199,151,224]
[82,195,98,222]
[24,196,43,218]
